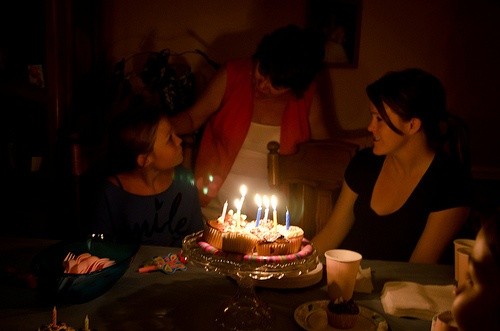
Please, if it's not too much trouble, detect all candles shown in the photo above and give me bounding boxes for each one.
[220,184,290,229]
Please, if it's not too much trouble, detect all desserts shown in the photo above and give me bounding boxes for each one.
[326,296,360,329]
[203,211,304,256]
[47,322,76,331]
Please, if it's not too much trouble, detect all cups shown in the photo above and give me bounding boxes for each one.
[431,311,461,331]
[325,249,362,300]
[456,248,471,287]
[454,239,477,281]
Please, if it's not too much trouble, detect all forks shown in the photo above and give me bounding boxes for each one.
[62,252,77,267]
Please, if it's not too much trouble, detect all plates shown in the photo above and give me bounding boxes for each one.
[294,300,388,331]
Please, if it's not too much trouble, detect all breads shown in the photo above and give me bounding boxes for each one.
[354,265,374,293]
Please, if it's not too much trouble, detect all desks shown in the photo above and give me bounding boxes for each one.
[0,240,457,331]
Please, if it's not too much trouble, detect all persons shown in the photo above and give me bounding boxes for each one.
[87,105,205,247]
[309,67,479,267]
[454,215,500,331]
[165,23,358,244]
